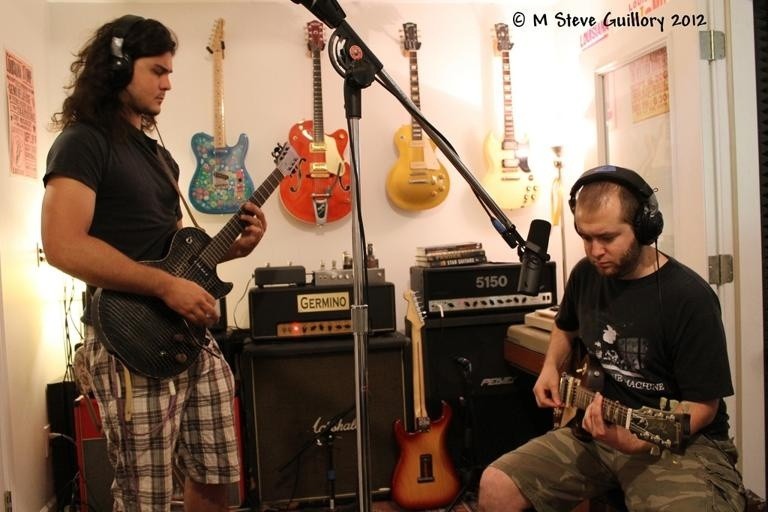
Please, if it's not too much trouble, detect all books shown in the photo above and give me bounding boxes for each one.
[415,240,487,270]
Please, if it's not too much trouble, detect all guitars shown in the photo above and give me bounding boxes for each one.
[386,21,450,211]
[391,288,462,509]
[484,23,537,211]
[553,353,690,464]
[278,20,353,224]
[188,18,254,214]
[89,143,304,381]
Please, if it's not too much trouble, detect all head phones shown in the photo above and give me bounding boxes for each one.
[569,165,663,246]
[103,15,144,90]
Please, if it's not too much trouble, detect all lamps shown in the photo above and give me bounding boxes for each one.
[547,136,569,293]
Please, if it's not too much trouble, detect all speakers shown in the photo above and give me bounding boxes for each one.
[404,310,554,493]
[240,331,416,506]
[72,397,245,512]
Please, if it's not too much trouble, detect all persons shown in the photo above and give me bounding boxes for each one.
[475,164,753,511]
[39,14,267,511]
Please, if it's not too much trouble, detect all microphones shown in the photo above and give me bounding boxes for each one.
[456,356,470,365]
[517,219,551,297]
